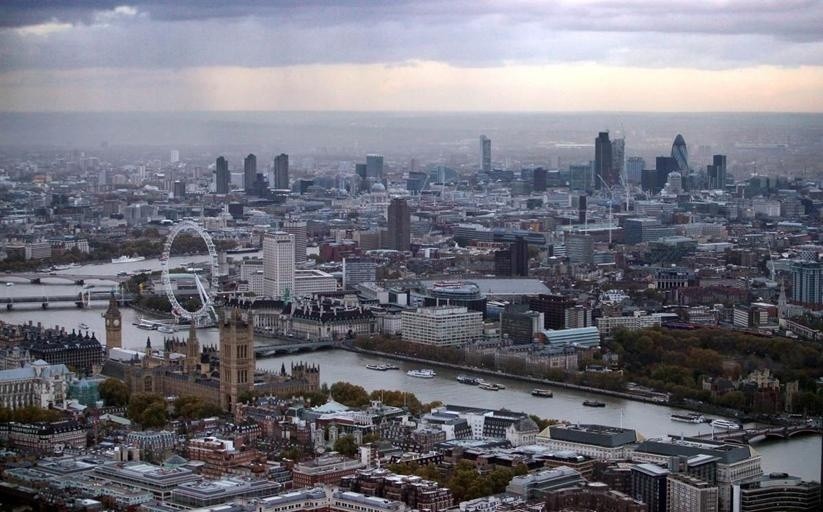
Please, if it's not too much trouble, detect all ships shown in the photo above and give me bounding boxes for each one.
[671,413,705,423]
[456,375,484,385]
[710,419,739,429]
[406,368,436,378]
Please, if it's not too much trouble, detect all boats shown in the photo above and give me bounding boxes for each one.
[583,401,605,407]
[532,388,552,397]
[366,363,399,371]
[480,382,505,391]
